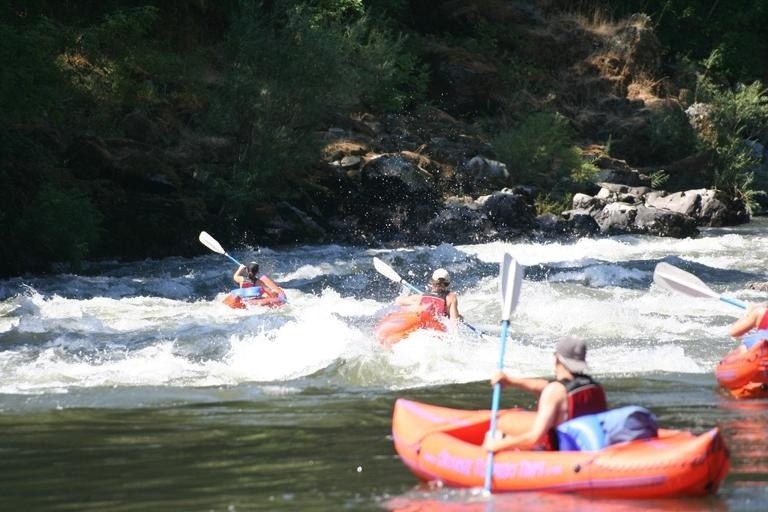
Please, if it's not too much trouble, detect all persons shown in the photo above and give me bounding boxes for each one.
[483,334,609,453]
[233,260,279,299]
[728,301,768,347]
[394,267,465,323]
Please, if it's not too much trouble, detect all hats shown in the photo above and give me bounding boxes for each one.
[246,262,259,273]
[554,337,589,374]
[432,268,451,284]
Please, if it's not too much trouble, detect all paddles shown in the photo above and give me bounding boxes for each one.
[653,262,747,309]
[373,256,483,343]
[483,252,524,498]
[198,231,289,304]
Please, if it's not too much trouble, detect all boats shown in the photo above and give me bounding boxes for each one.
[392,398,730,497]
[223,287,285,310]
[374,311,445,342]
[716,340,768,401]
